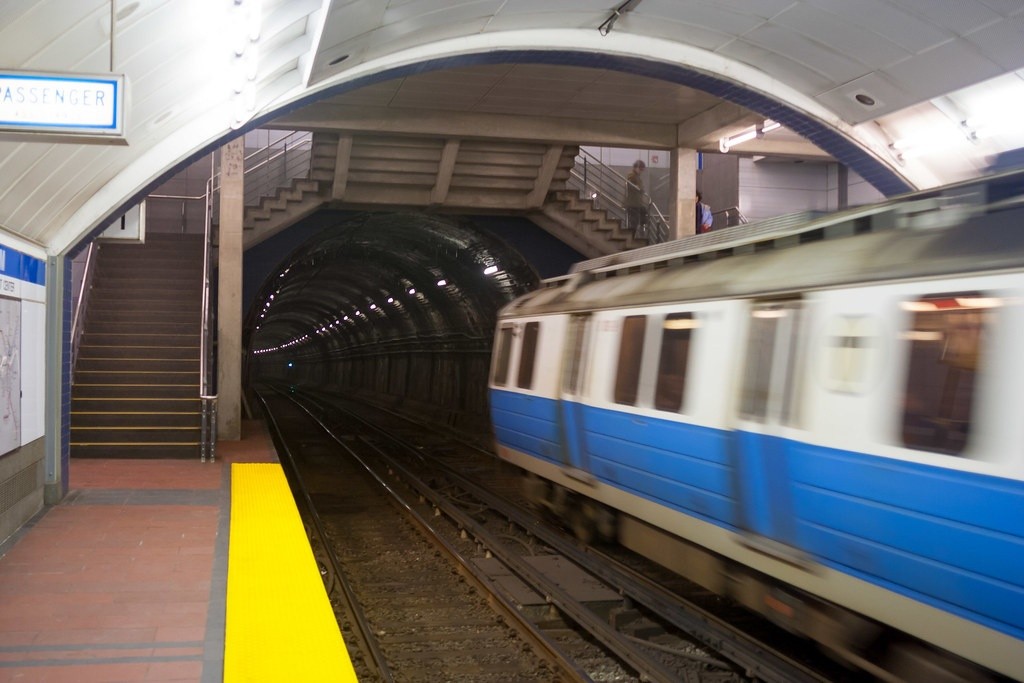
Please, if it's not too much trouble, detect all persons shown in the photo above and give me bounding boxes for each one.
[696,191,702,234]
[623,160,646,249]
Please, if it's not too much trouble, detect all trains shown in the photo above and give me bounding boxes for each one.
[488,160,1024,681]
